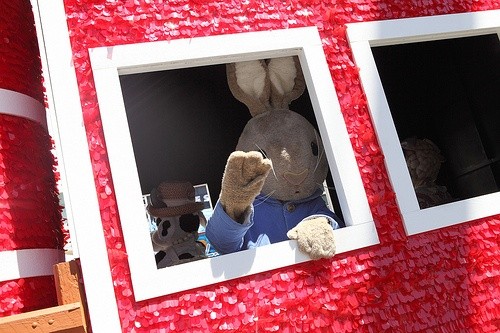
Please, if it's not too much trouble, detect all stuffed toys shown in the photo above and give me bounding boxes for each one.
[148,213,202,248]
[401,138,452,209]
[202,56,344,262]
